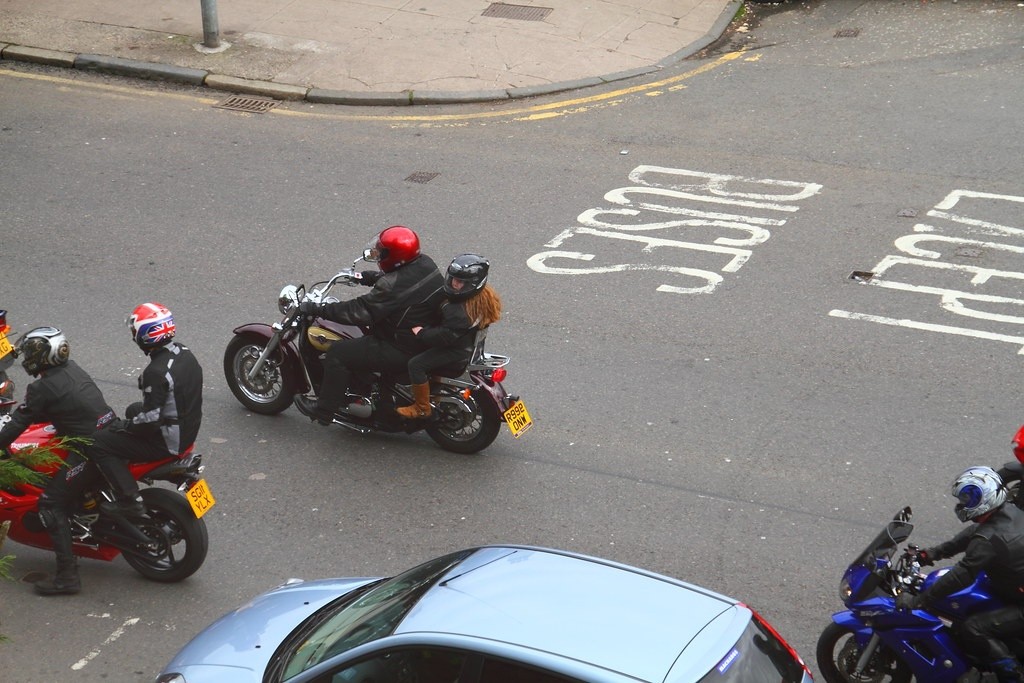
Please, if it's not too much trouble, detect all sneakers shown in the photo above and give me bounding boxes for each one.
[99,492,147,517]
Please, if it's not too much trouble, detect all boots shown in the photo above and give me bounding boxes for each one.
[397,382,432,419]
[33,556,82,595]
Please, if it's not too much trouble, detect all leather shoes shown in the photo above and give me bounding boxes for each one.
[294,393,333,425]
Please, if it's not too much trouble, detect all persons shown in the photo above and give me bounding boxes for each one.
[0,327,120,595]
[896,464,1024,683]
[294,226,501,425]
[82,301,202,517]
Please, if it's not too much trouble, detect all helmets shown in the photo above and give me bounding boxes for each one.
[125,302,176,356]
[442,254,490,302]
[13,326,69,376]
[951,466,1011,523]
[370,225,421,272]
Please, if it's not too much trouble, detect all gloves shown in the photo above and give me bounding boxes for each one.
[300,301,319,317]
[359,270,379,286]
[895,592,918,610]
[110,419,133,435]
[916,548,933,567]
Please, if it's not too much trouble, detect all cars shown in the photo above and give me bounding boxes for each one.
[154,540,814,682]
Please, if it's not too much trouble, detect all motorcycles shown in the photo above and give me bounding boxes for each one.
[221,248,536,458]
[814,505,1024,682]
[0,310,21,414]
[0,381,218,582]
